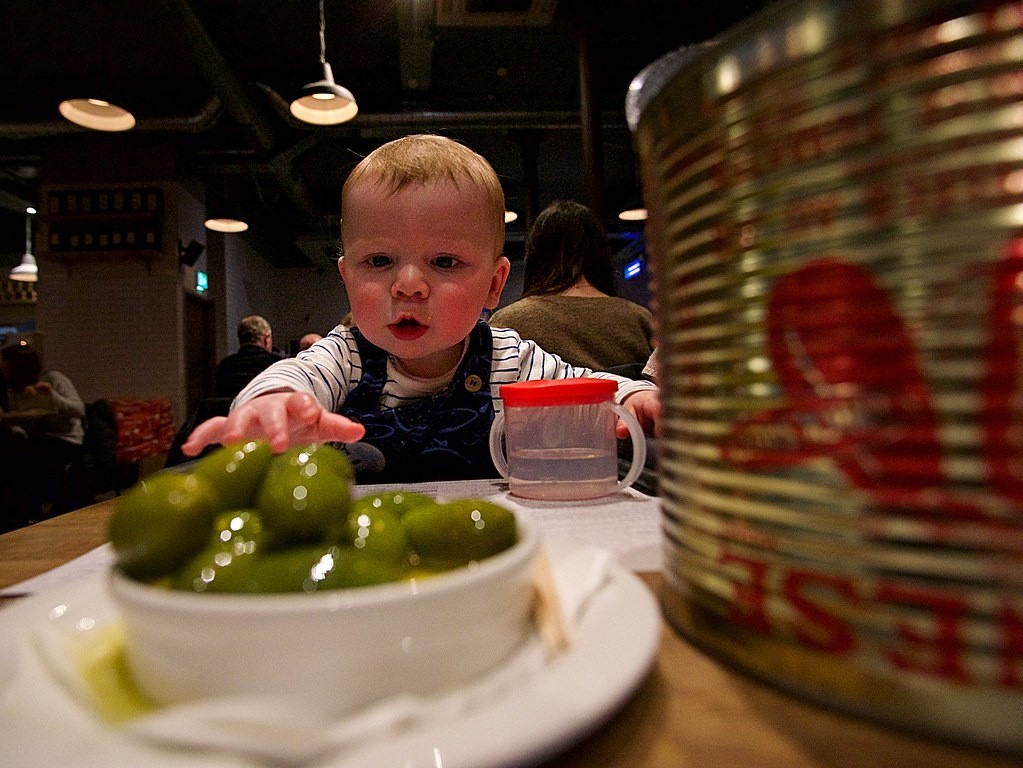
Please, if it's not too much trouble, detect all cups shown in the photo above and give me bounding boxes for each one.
[490,378,646,501]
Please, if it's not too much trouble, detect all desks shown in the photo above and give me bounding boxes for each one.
[0,457,1023,768]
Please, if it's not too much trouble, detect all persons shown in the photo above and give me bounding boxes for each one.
[181,132,660,484]
[194,315,291,437]
[486,197,661,385]
[1,335,86,539]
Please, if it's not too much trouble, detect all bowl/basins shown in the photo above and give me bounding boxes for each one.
[107,500,540,707]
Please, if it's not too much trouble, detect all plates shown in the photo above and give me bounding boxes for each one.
[0,532,663,768]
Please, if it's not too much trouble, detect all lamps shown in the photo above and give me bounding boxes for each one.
[289,0,358,126]
[198,175,250,235]
[179,240,206,266]
[7,216,38,283]
